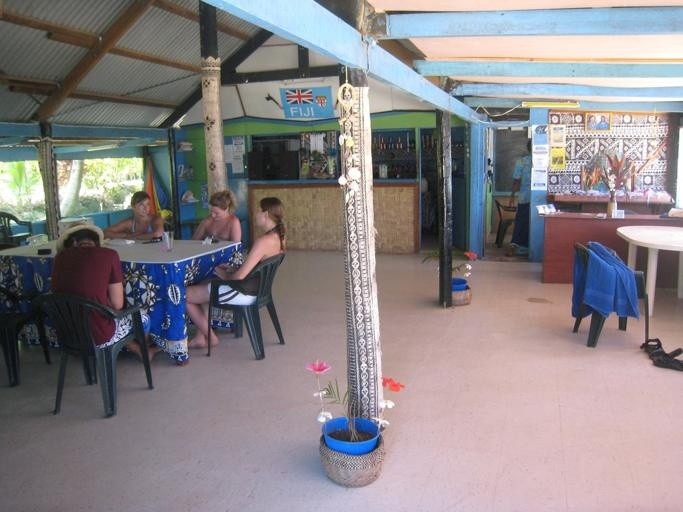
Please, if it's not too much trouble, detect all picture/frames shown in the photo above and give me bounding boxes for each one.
[584,110,613,132]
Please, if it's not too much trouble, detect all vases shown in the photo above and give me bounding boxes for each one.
[451,286,472,306]
[607,202,617,217]
[451,278,468,290]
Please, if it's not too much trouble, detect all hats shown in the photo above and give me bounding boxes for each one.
[55,219,104,251]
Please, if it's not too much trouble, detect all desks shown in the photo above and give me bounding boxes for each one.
[616,225,683,316]
[0,238,243,367]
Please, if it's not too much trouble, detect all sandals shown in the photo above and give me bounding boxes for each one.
[641,338,682,370]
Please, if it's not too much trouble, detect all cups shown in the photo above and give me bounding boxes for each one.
[162,231,175,256]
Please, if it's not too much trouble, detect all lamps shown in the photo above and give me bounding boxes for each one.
[522,101,580,109]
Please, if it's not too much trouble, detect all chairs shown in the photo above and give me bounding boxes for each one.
[30,287,154,418]
[206,253,285,360]
[1,211,33,252]
[1,281,52,387]
[570,240,649,347]
[494,198,518,247]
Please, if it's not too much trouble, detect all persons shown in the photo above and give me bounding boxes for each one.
[103,192,164,240]
[190,190,242,276]
[597,116,610,130]
[587,116,597,130]
[510,140,532,256]
[51,220,163,364]
[184,197,286,350]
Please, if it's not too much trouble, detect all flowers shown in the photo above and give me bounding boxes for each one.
[303,360,406,441]
[421,249,477,279]
[581,153,636,202]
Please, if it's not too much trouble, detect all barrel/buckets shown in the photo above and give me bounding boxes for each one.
[57,216,93,236]
[451,276,470,288]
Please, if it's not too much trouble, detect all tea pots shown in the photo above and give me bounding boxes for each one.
[451,157,458,176]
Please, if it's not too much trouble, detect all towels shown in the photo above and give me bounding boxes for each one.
[571,239,640,320]
